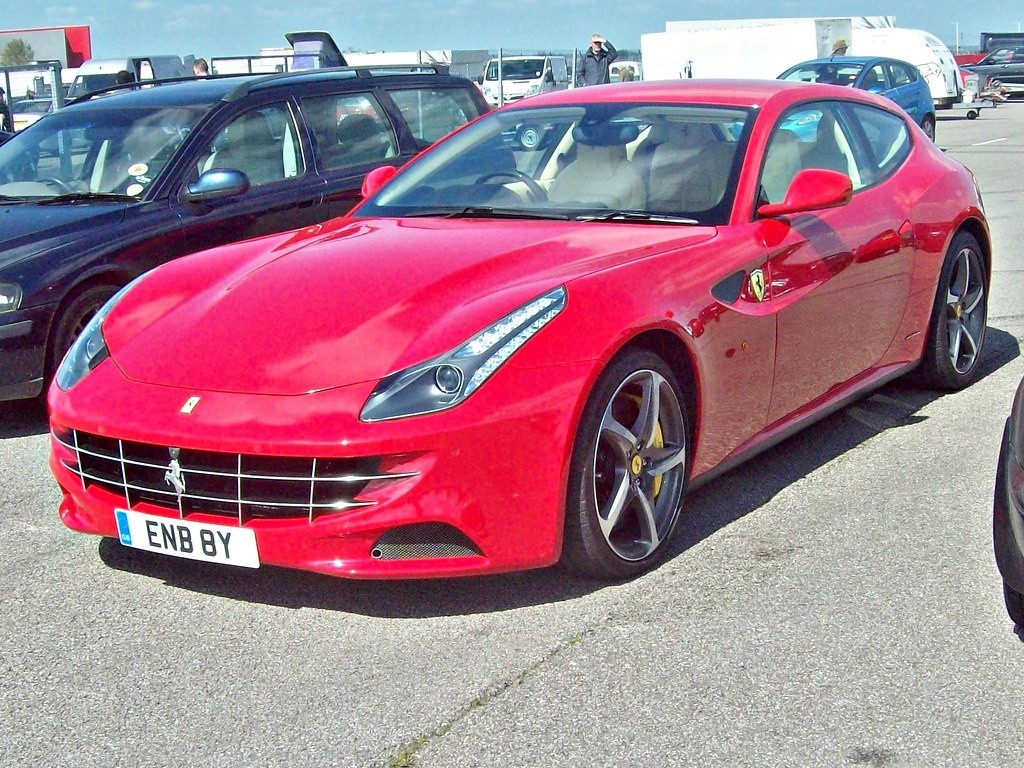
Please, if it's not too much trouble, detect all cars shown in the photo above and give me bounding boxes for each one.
[337,87,438,133]
[992,376,1024,627]
[0,97,95,156]
[958,46,1024,103]
[733,47,937,164]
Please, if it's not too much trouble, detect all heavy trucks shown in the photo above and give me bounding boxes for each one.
[641,18,964,119]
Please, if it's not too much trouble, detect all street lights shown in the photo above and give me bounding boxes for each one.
[952,22,959,56]
[1014,20,1020,31]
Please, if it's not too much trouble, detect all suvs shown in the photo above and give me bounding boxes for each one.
[0,64,517,414]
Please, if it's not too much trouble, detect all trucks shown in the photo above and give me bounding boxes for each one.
[478,55,569,109]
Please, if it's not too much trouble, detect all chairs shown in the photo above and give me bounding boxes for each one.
[716,127,802,209]
[323,114,389,168]
[202,111,284,191]
[545,141,646,210]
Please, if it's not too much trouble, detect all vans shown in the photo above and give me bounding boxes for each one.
[67,54,185,97]
[608,61,642,84]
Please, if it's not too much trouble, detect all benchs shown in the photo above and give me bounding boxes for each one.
[646,122,845,199]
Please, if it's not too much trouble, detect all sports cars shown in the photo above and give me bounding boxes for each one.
[45,77,991,583]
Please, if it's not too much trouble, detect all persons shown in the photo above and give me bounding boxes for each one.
[830,39,848,57]
[193,58,210,80]
[619,65,634,82]
[577,33,619,86]
[116,70,132,85]
[0,87,11,130]
